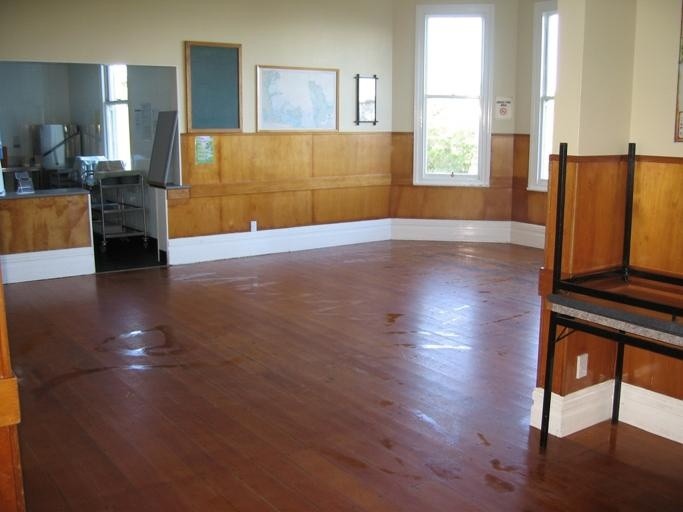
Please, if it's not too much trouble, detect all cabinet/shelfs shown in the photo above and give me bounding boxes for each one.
[49,170,149,254]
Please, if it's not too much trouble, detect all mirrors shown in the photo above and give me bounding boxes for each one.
[354,74,379,125]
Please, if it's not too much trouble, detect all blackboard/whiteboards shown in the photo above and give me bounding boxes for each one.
[256,64,339,133]
[185,41,243,134]
